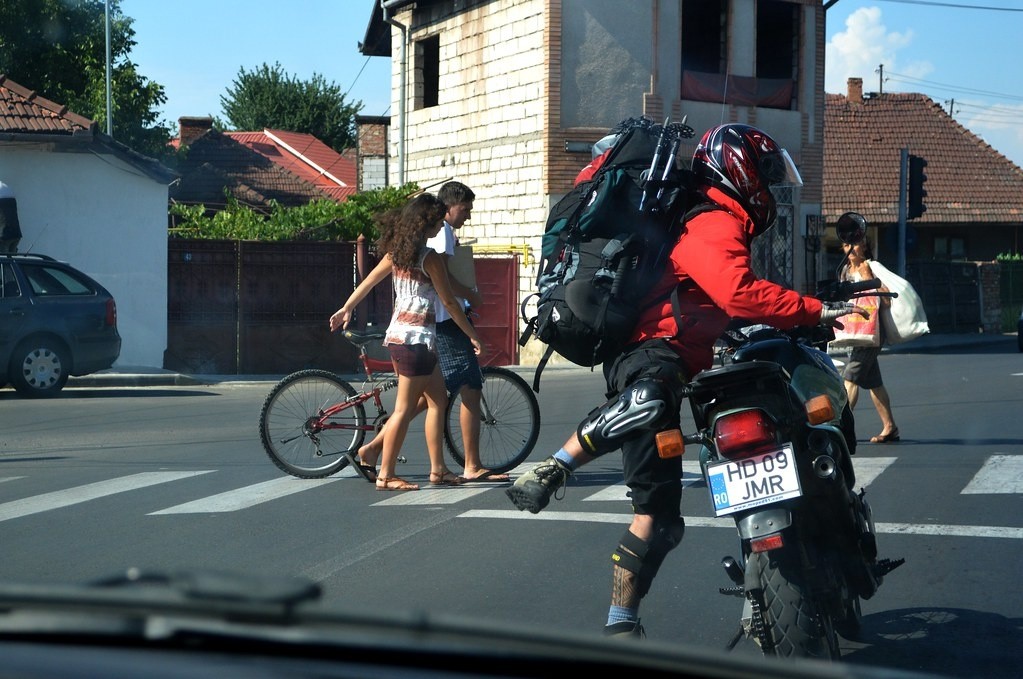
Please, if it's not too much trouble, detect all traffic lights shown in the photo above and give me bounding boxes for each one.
[908,157,928,219]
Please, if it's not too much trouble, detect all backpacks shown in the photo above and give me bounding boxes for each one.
[534,117,751,368]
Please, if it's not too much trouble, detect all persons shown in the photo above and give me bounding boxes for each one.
[346,181,509,482]
[504,123,870,638]
[842,242,900,443]
[329,192,486,488]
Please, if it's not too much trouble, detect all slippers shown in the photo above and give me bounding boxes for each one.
[346,453,377,483]
[465,471,510,482]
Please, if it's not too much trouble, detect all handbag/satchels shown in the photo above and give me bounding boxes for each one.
[830,259,930,348]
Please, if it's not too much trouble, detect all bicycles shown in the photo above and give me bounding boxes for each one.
[258,328,541,481]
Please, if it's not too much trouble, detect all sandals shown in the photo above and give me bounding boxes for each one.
[870,427,900,443]
[429,471,469,485]
[375,474,419,490]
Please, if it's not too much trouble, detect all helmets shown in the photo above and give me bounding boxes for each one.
[691,123,786,238]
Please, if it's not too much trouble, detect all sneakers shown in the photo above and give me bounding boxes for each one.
[505,455,579,514]
[600,618,647,641]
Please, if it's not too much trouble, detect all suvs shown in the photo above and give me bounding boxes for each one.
[0,251,123,400]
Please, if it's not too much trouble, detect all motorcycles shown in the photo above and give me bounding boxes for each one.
[653,210,907,663]
